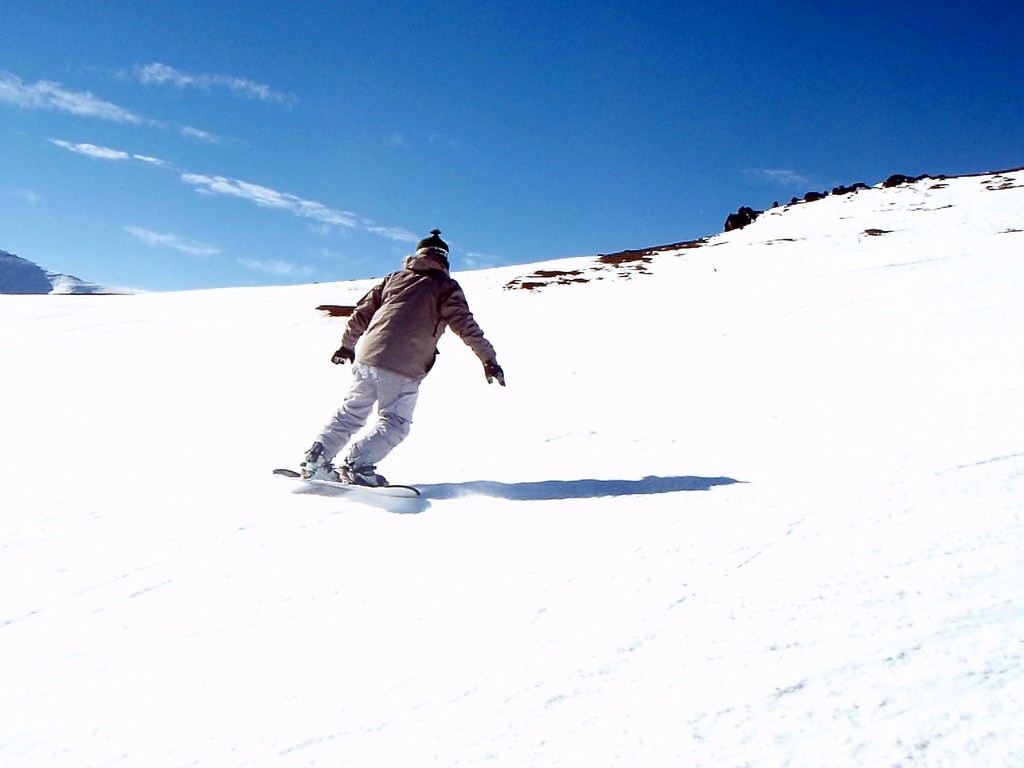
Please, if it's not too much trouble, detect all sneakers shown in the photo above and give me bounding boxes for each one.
[339,456,389,487]
[300,441,339,482]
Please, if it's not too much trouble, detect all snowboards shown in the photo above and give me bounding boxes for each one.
[274,467,421,498]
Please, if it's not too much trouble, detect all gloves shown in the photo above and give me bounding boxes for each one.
[332,346,355,364]
[483,356,505,387]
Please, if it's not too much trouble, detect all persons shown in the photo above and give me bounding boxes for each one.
[300,229,506,485]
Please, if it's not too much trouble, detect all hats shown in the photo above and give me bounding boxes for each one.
[416,229,449,253]
